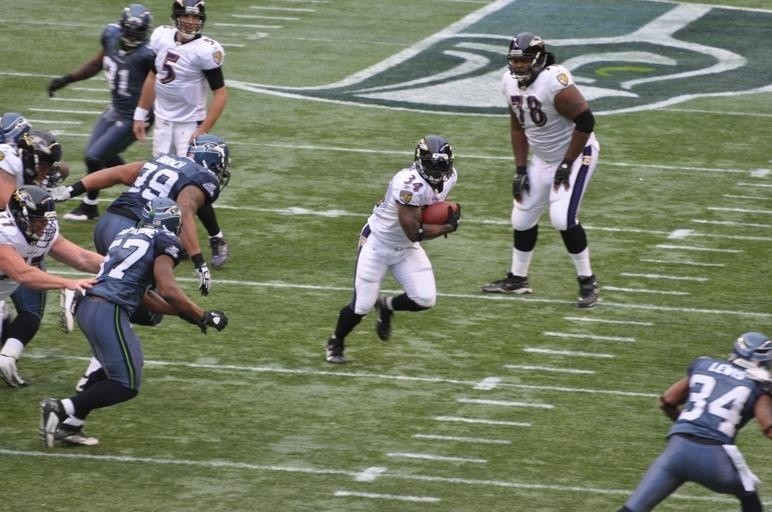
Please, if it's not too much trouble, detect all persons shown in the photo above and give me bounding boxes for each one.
[481,31,602,309]
[48,0,230,270]
[616,330,772,512]
[0,112,232,448]
[324,133,463,365]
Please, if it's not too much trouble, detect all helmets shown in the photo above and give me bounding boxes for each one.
[728,332,772,370]
[0,113,62,248]
[507,31,547,89]
[169,0,205,39]
[186,133,230,191]
[415,135,453,186]
[117,4,151,47]
[140,198,182,235]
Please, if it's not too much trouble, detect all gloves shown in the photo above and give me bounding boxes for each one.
[48,75,71,95]
[45,180,87,203]
[191,253,212,296]
[177,310,225,333]
[553,160,571,191]
[510,167,530,202]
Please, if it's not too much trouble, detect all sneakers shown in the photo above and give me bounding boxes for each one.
[0,354,28,389]
[577,275,600,308]
[325,338,345,363]
[210,238,227,268]
[483,273,533,295]
[60,287,80,331]
[39,399,99,450]
[62,204,99,220]
[375,296,393,342]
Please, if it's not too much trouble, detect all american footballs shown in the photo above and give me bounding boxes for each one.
[423,202,458,224]
